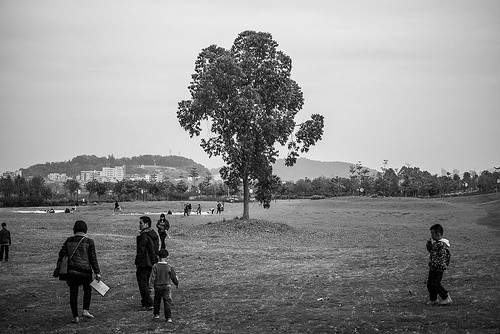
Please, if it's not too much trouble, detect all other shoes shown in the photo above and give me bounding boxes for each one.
[72,318,79,323]
[438,297,452,305]
[82,311,94,319]
[426,300,438,305]
[154,316,160,319]
[167,318,172,323]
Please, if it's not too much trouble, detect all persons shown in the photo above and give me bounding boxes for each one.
[65,205,76,213]
[184,203,191,216]
[58,220,101,322]
[0,222,11,261]
[211,201,224,214]
[151,250,179,322]
[115,201,121,212]
[50,209,53,213]
[198,204,201,214]
[134,216,159,311]
[426,223,452,305]
[157,214,169,249]
[75,197,85,206]
